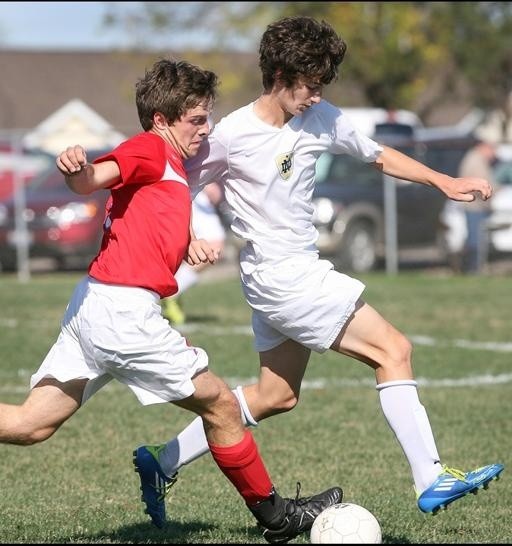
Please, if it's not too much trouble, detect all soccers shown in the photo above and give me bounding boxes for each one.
[310,503,383,545]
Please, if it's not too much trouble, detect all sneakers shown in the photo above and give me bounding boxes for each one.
[133,442,178,530]
[415,462,505,516]
[255,486,343,545]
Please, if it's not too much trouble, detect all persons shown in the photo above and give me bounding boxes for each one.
[443,124,504,274]
[130,16,505,528]
[1,57,345,544]
[165,182,228,324]
